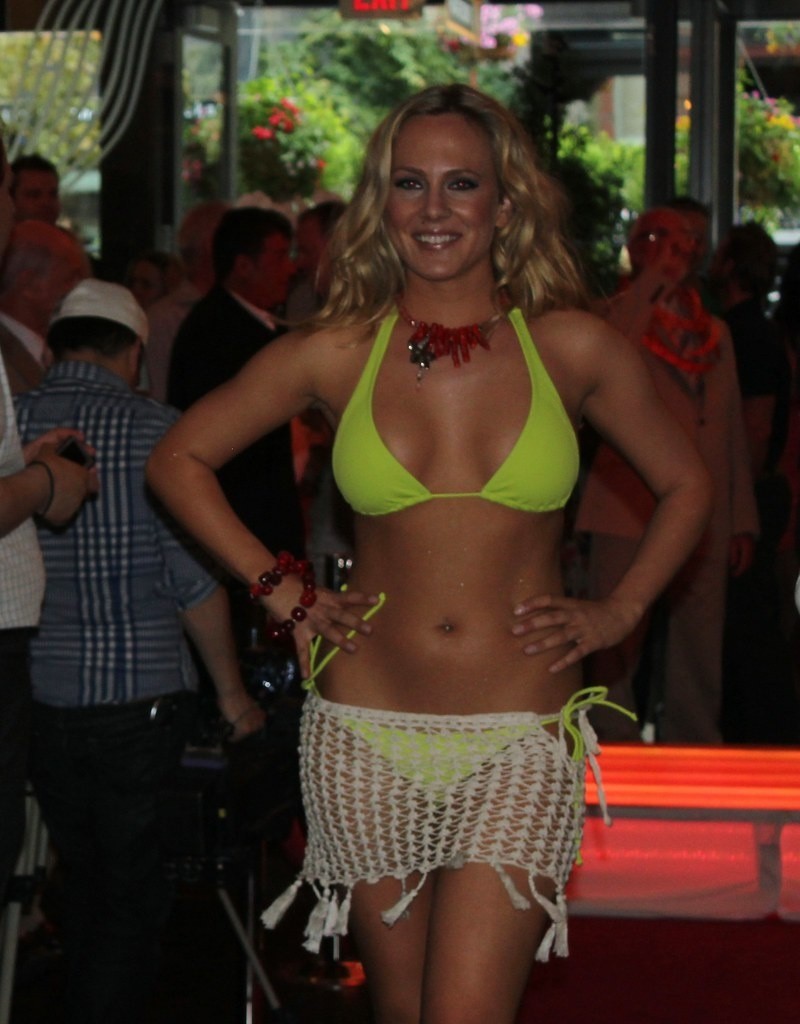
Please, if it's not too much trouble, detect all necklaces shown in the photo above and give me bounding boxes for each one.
[392,288,505,382]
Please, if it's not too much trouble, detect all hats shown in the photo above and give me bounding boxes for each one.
[51,279,148,346]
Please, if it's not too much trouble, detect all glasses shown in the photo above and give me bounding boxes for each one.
[637,228,670,240]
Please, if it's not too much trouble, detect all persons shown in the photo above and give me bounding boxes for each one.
[165,208,304,644]
[147,80,718,1024]
[566,202,755,752]
[2,142,800,387]
[20,279,257,1023]
[0,355,102,922]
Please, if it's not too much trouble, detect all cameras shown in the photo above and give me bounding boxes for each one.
[55,435,95,470]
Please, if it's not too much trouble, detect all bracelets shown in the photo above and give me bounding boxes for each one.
[248,554,317,640]
[26,460,55,519]
